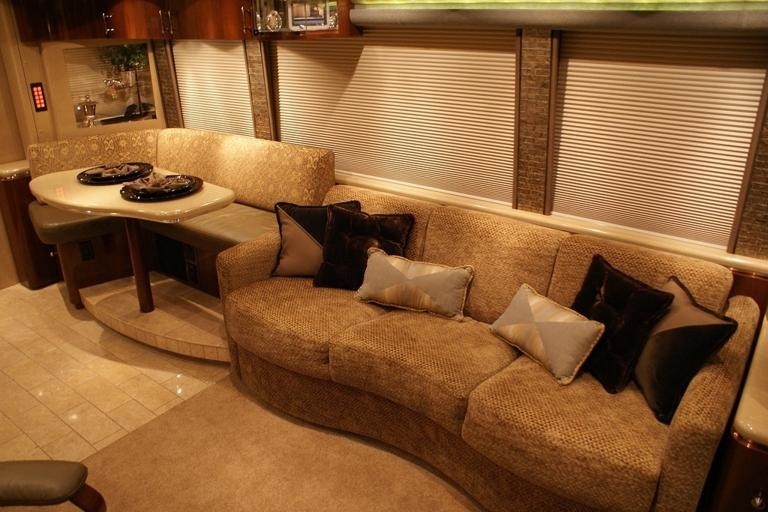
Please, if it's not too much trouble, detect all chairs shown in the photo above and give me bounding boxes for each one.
[0,460,106,512]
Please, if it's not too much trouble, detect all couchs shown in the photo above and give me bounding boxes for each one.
[215,184,761,511]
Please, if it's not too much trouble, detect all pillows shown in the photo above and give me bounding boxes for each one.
[271,200,474,322]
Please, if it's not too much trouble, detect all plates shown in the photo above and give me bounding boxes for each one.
[120,174,203,202]
[77,162,153,185]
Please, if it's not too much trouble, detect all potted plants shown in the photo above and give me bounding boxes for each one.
[107,45,149,88]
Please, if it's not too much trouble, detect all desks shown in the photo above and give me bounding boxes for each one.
[29,166,236,313]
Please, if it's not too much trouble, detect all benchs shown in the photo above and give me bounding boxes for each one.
[27,128,335,309]
